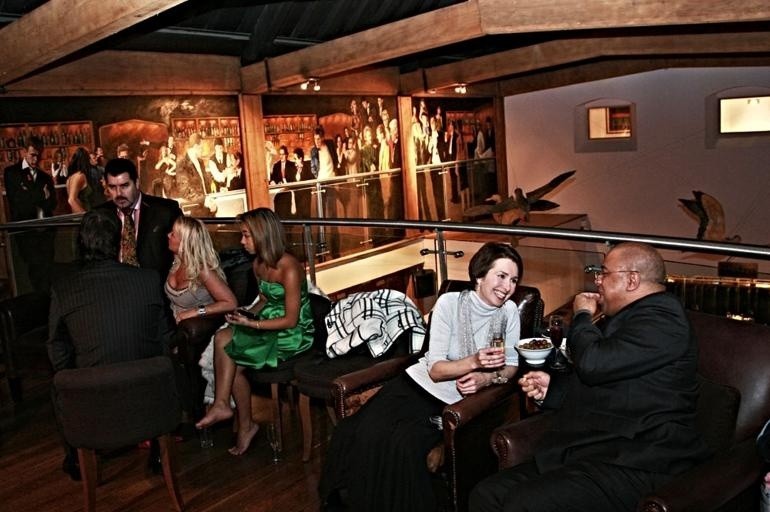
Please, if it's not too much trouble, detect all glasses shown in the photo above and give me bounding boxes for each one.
[594,270,639,280]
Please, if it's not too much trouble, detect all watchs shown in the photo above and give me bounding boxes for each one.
[493,371,508,384]
[197,303,206,316]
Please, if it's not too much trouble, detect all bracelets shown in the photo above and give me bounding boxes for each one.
[257,320,261,329]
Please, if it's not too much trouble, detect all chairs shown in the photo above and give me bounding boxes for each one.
[1,291,53,418]
[49,358,184,510]
[299,335,404,463]
[242,293,330,462]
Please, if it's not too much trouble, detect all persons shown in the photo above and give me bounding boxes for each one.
[140,136,177,154]
[164,216,237,346]
[468,242,699,512]
[196,207,316,455]
[117,144,129,160]
[151,146,177,198]
[177,133,217,213]
[209,138,233,192]
[47,209,178,482]
[89,152,102,181]
[317,242,524,512]
[336,97,498,247]
[4,136,57,294]
[97,158,183,271]
[292,148,316,182]
[52,150,68,185]
[265,140,276,181]
[269,145,296,184]
[66,147,93,260]
[227,152,246,191]
[96,147,105,159]
[310,128,340,262]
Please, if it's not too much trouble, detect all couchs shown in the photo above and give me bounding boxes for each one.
[169,269,255,423]
[487,304,770,512]
[335,280,543,510]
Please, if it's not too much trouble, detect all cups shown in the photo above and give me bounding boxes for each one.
[492,332,505,357]
[550,316,566,369]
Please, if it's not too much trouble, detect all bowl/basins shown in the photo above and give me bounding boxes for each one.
[514,338,554,365]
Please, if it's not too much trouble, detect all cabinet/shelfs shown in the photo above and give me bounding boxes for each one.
[0,119,97,225]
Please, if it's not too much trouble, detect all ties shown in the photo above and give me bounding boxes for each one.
[121,208,139,267]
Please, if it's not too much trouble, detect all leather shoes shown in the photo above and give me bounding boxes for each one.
[146,454,162,476]
[62,455,80,481]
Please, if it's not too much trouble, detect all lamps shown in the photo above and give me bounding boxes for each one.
[305,76,320,91]
[300,76,319,90]
[455,82,468,95]
